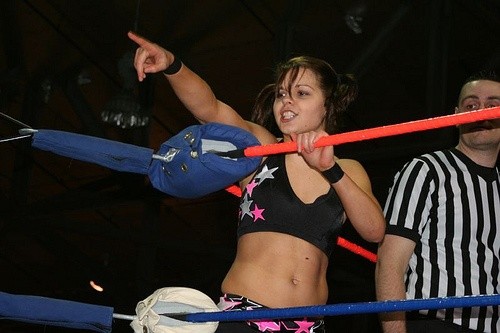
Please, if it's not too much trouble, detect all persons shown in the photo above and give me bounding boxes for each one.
[127,31,387,333]
[375,75,500,333]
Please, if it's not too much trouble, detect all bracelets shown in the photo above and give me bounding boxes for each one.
[321,162,344,184]
[163,55,182,75]
